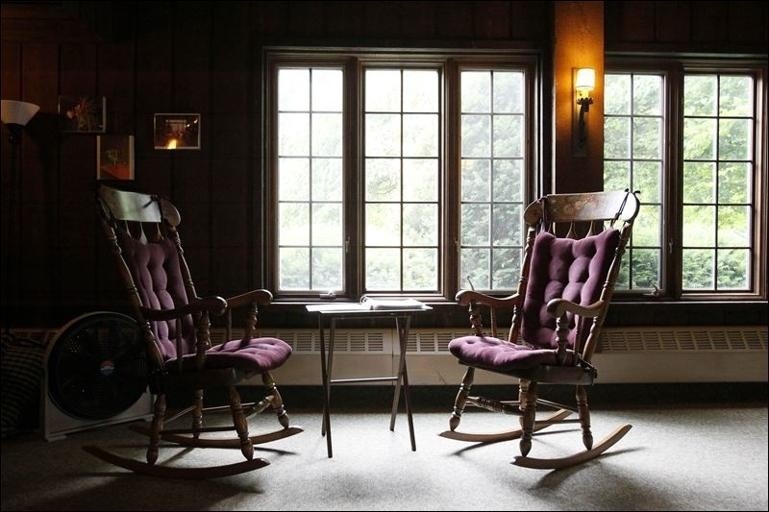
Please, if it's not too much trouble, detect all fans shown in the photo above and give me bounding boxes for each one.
[40,311,157,443]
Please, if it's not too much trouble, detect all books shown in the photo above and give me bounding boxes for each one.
[304,296,434,311]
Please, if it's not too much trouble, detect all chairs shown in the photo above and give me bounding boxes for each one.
[437,188,640,470]
[82,184,303,483]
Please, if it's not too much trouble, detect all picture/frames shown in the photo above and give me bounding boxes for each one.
[97,135,135,180]
[58,96,107,133]
[153,113,202,150]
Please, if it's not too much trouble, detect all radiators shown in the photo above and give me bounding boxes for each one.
[209,326,768,385]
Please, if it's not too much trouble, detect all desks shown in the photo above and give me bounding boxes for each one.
[317,306,433,460]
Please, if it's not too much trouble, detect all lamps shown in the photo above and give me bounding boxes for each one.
[571,66,595,158]
[0,100,41,145]
